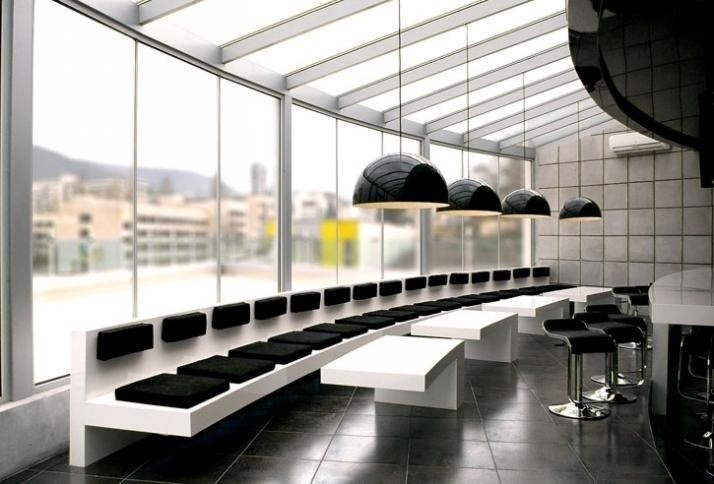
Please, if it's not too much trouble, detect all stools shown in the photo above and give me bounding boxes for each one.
[676,329,714,476]
[541,283,654,419]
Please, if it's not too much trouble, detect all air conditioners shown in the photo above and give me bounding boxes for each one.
[609,130,672,154]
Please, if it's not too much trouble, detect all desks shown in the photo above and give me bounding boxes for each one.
[319,285,612,411]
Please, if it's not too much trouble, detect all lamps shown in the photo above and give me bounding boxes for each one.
[557,102,601,218]
[503,73,553,219]
[435,22,502,218]
[350,0,450,211]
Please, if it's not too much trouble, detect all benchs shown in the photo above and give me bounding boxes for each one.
[68,265,577,469]
